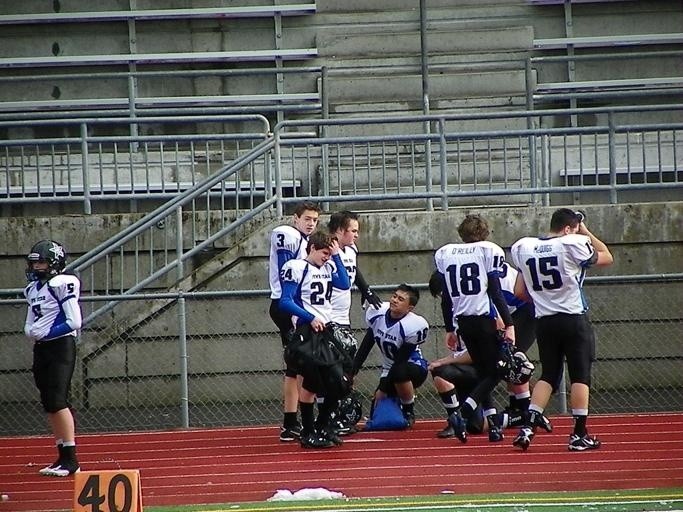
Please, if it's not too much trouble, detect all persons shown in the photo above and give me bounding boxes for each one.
[328,212,382,434]
[427,336,552,439]
[24,241,81,477]
[434,214,516,443]
[428,263,537,428]
[511,209,613,451]
[352,284,429,427]
[269,201,322,442]
[280,232,351,447]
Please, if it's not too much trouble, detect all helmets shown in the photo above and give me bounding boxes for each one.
[496,341,535,385]
[24,240,66,281]
[341,401,362,422]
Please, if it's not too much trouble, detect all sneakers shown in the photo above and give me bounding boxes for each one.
[313,422,342,443]
[300,432,334,448]
[568,433,600,451]
[512,426,534,450]
[449,409,468,444]
[488,426,504,441]
[404,409,415,428]
[39,462,62,475]
[279,422,304,442]
[523,408,553,431]
[337,420,350,436]
[438,423,454,437]
[50,463,81,476]
